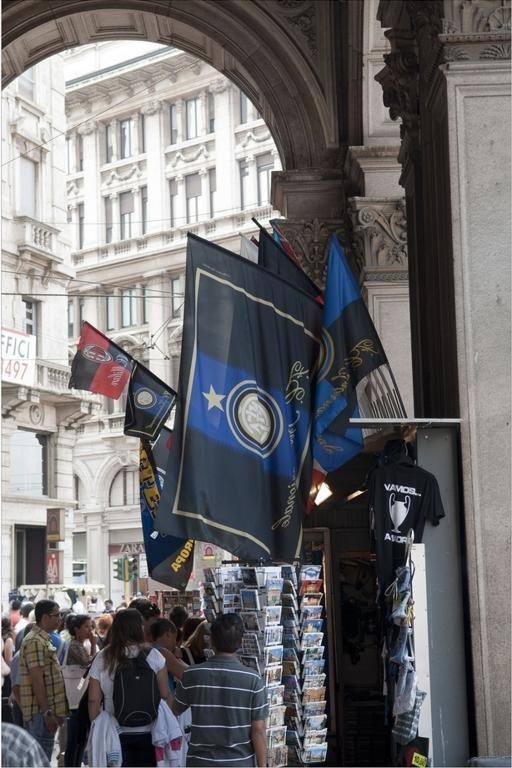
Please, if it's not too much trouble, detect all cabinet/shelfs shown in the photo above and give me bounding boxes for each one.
[156,590,202,619]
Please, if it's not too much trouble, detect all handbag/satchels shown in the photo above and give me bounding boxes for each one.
[61,664,90,709]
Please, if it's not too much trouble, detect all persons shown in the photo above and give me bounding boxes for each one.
[3,588,270,767]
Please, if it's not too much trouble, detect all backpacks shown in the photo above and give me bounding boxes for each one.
[113,647,161,727]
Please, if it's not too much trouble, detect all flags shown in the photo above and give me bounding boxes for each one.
[66,227,401,596]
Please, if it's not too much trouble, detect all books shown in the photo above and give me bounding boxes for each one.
[197,561,330,766]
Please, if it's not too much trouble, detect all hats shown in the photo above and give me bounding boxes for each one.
[29,610,37,624]
